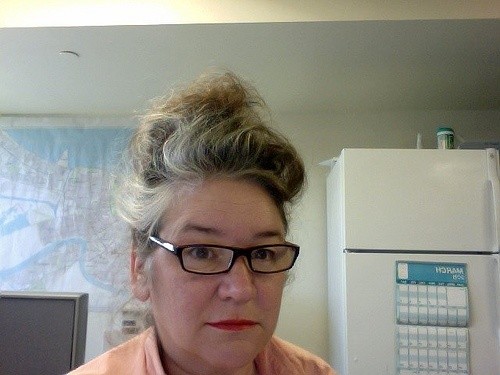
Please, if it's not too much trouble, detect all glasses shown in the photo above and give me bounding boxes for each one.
[148,236,300,275]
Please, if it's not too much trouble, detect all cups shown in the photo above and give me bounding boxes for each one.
[436,127,455,149]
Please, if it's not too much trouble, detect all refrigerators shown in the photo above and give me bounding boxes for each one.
[326,148,500,375]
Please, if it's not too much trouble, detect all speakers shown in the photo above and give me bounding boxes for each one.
[0,291,89,375]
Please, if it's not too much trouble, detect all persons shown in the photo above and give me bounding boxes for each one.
[62,73,338,375]
